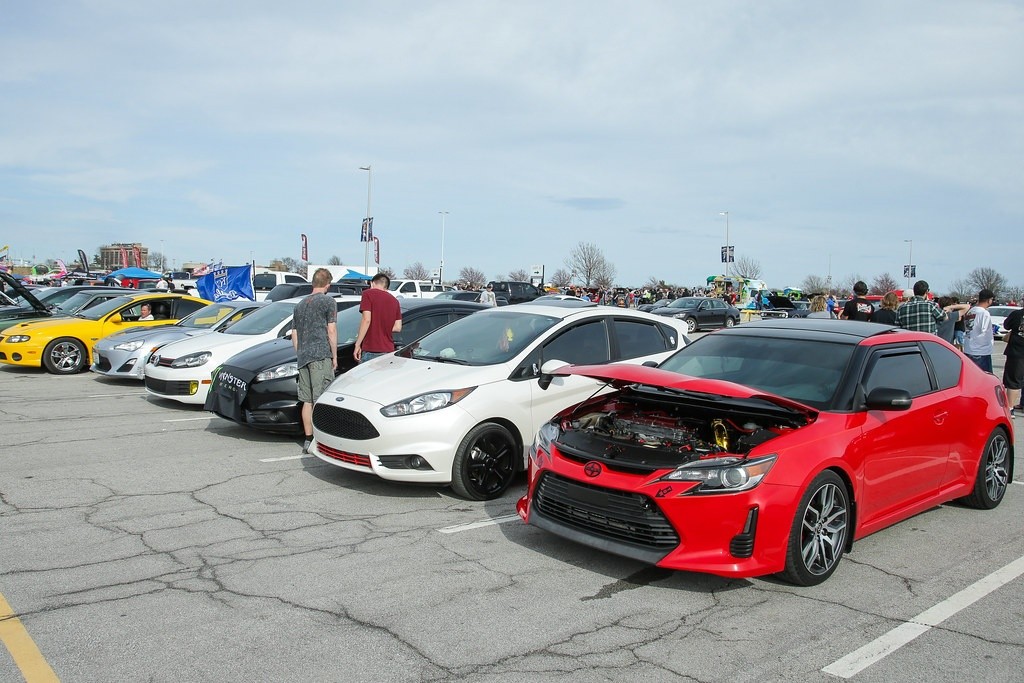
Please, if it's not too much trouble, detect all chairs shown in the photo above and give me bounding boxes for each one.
[153,303,170,320]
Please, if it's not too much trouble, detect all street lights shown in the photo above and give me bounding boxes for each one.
[904,240,912,289]
[359,166,372,275]
[437,210,449,284]
[720,210,729,276]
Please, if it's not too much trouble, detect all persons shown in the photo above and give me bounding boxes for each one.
[291,268,338,454]
[479,283,497,306]
[156,277,175,293]
[1002,307,1024,418]
[842,281,875,322]
[138,303,155,321]
[873,291,898,325]
[925,291,979,352]
[963,289,993,374]
[0,264,134,288]
[353,273,402,365]
[752,291,854,320]
[537,283,736,308]
[445,281,488,299]
[894,280,948,336]
[990,295,1024,307]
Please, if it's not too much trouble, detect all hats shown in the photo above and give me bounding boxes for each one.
[979,288,995,299]
[487,283,493,288]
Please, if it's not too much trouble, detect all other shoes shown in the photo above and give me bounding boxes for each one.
[303,439,311,455]
[1014,404,1023,409]
[1011,409,1015,419]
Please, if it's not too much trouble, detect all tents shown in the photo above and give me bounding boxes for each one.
[107,267,164,278]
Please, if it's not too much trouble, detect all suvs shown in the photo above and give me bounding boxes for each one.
[264,283,372,303]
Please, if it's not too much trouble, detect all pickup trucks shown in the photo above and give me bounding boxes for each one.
[453,280,547,305]
[833,291,935,315]
[163,271,199,291]
[388,279,447,299]
[187,270,311,301]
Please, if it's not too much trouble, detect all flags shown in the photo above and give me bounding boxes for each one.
[196,264,255,303]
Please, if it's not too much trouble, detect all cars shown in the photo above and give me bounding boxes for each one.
[987,306,1024,338]
[637,296,741,334]
[145,292,400,405]
[87,300,272,380]
[516,317,1016,587]
[766,296,812,318]
[305,299,705,500]
[0,271,137,330]
[532,296,586,321]
[0,291,242,375]
[204,296,496,434]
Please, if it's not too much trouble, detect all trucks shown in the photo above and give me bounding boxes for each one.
[707,277,774,309]
